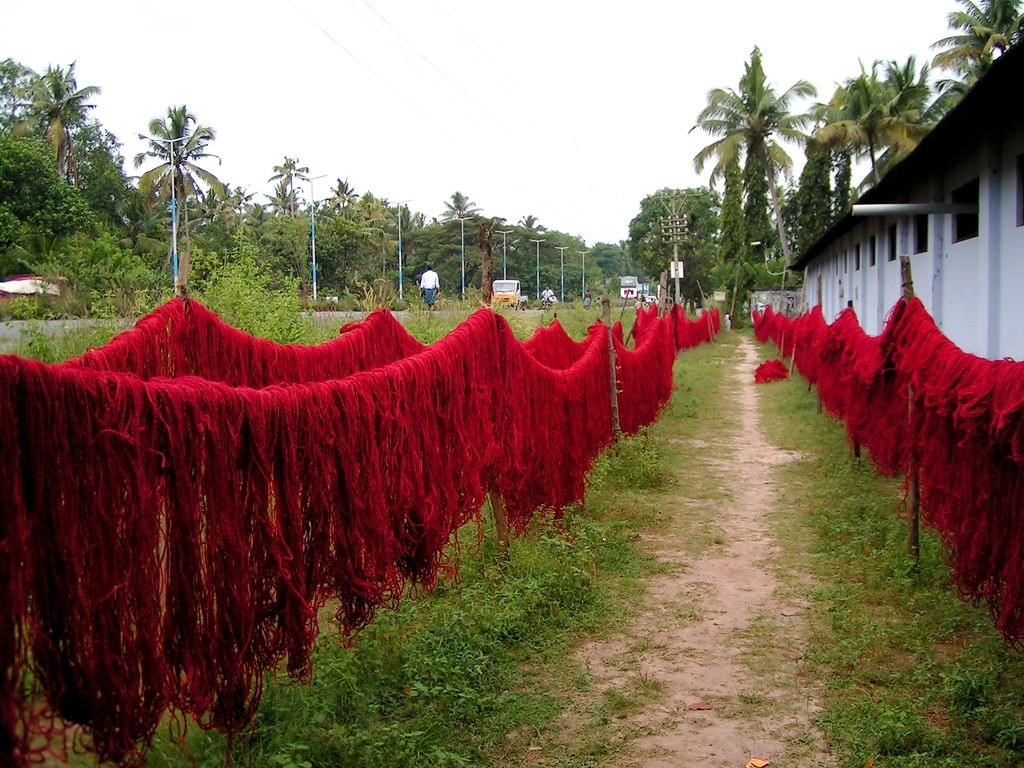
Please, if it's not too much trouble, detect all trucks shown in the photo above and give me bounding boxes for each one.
[492,280,528,310]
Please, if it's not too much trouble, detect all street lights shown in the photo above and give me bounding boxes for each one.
[554,246,570,303]
[494,230,515,280]
[137,134,194,297]
[529,238,547,299]
[386,199,410,300]
[577,250,589,300]
[294,172,330,300]
[450,216,475,301]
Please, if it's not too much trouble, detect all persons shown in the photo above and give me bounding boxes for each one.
[419,262,440,306]
[542,286,554,300]
[583,290,591,307]
